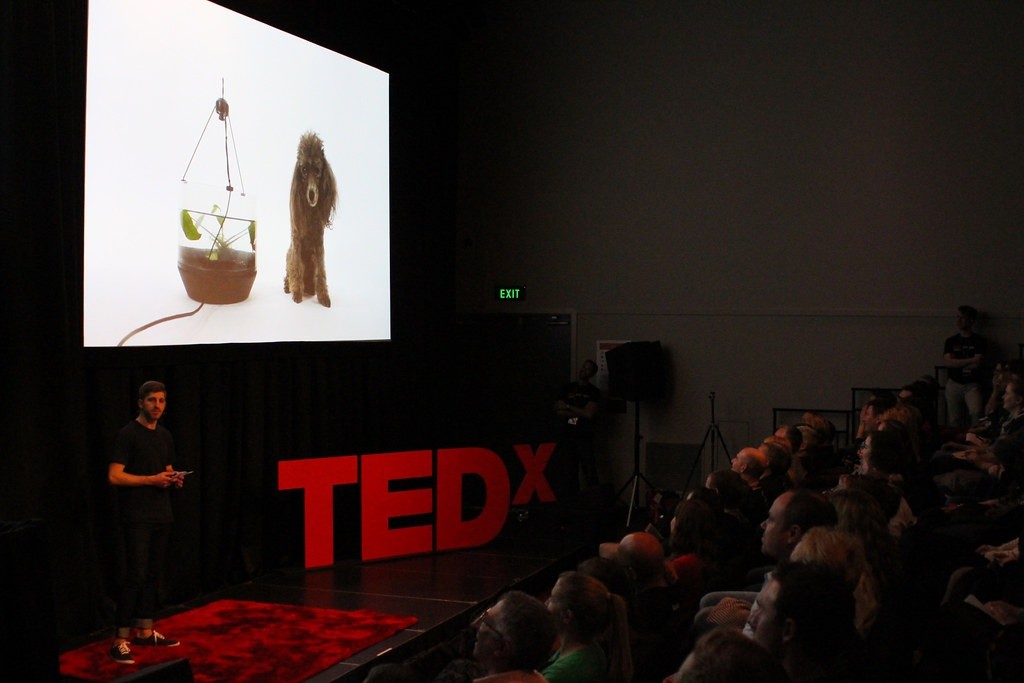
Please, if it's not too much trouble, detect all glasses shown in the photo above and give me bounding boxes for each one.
[480,606,506,644]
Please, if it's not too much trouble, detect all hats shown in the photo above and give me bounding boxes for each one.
[598,531,665,573]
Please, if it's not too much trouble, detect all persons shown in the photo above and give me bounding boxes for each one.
[542,573,634,683]
[580,359,1024,683]
[466,592,558,683]
[362,664,416,683]
[551,359,603,502]
[939,303,989,430]
[108,380,180,665]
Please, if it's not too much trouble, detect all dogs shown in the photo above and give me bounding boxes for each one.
[283,131,338,308]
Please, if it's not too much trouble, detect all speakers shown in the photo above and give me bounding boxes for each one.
[606,340,674,400]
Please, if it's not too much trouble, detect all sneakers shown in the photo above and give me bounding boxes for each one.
[109,642,135,663]
[131,629,180,646]
